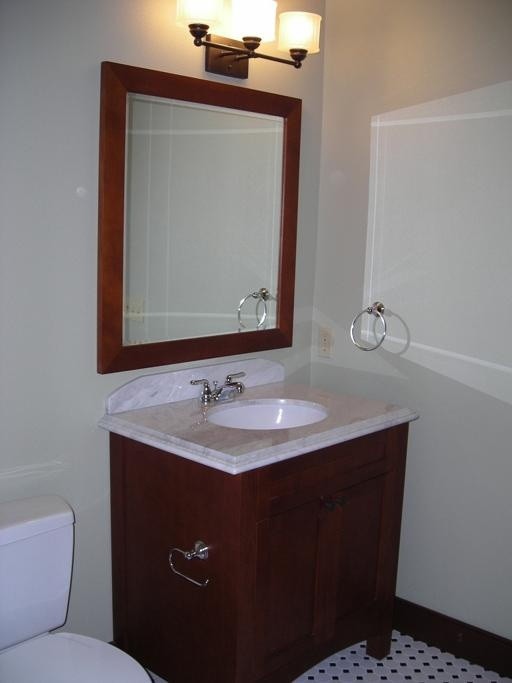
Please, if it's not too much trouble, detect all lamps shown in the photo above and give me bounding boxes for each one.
[176,0,322,80]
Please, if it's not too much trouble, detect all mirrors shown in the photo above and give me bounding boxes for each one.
[98,60,302,377]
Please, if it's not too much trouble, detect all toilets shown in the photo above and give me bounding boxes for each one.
[0,495,177,682]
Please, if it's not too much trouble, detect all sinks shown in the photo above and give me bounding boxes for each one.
[203,397,332,431]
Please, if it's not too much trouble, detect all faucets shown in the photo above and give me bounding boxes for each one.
[192,371,245,404]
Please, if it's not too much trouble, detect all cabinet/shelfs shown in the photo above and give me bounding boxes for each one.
[108,422,409,683]
